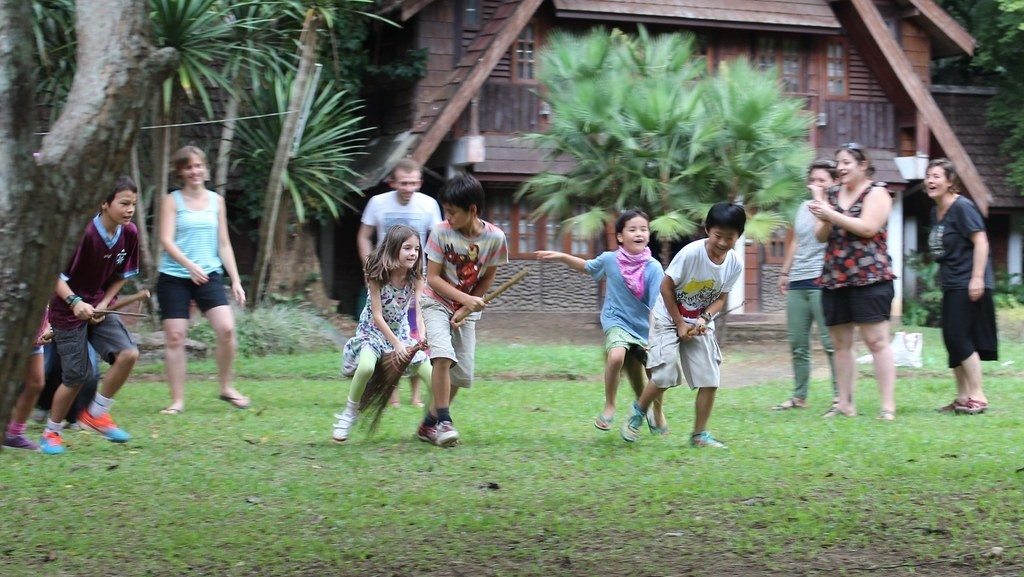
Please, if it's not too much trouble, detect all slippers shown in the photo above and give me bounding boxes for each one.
[219,393,251,409]
[770,398,808,410]
[876,409,896,422]
[647,409,667,435]
[594,411,615,430]
[820,401,858,419]
[160,406,188,417]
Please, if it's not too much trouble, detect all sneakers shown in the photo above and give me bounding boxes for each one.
[38,430,65,455]
[689,431,728,449]
[622,402,645,442]
[79,409,132,442]
[414,421,460,447]
[0,432,40,452]
[332,409,357,441]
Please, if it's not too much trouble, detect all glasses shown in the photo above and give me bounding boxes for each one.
[813,159,838,168]
[840,143,869,160]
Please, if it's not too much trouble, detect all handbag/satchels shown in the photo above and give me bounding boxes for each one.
[893,332,923,369]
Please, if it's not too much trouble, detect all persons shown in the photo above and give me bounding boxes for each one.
[332,160,512,448]
[622,203,747,448]
[534,208,671,438]
[770,160,846,412]
[155,145,251,416]
[925,159,999,414]
[808,142,898,421]
[6,175,143,454]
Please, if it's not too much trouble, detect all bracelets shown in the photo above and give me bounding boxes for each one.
[69,297,82,310]
[780,271,789,276]
[701,313,712,321]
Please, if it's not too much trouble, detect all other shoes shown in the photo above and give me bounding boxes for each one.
[935,398,988,414]
[391,399,425,409]
[63,421,82,431]
[28,412,46,433]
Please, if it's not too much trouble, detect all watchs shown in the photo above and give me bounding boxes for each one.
[65,293,78,304]
